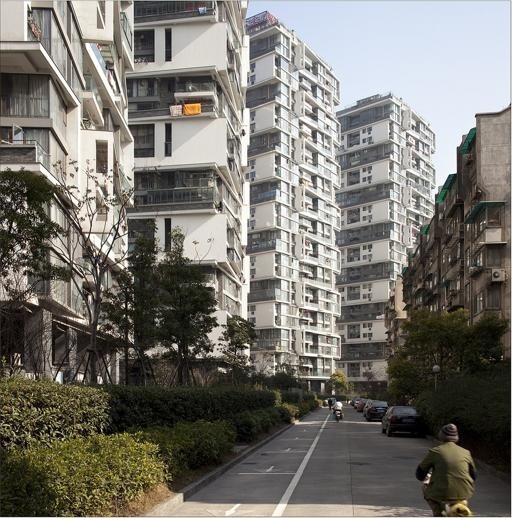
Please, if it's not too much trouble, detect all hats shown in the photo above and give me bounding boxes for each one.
[439,423,460,442]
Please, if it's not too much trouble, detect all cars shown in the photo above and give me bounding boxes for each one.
[380,405,428,439]
[349,397,391,422]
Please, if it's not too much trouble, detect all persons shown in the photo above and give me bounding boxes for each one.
[413,423,478,517]
[331,399,343,414]
[327,397,335,411]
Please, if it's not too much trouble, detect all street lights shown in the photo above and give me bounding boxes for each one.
[432,365,441,391]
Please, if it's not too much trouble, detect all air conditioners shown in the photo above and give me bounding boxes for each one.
[491,269,505,282]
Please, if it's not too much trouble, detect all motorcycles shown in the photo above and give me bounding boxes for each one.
[415,471,474,516]
[332,407,345,423]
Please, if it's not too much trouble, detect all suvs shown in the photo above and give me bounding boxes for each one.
[324,397,336,407]
